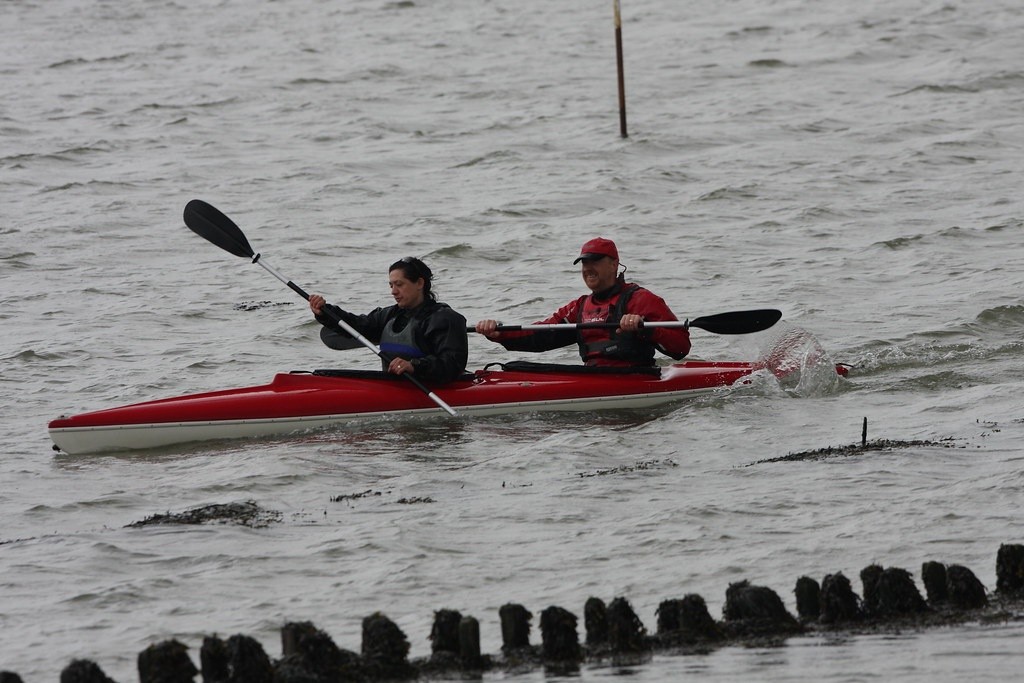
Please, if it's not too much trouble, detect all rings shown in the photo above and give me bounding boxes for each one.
[398,365,401,368]
[630,320,633,322]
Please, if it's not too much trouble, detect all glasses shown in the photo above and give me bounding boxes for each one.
[401,257,418,271]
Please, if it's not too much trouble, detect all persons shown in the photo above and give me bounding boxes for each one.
[476,237,692,370]
[308,257,469,387]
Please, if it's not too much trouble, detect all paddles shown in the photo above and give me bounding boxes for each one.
[184,196,465,420]
[465,309,783,337]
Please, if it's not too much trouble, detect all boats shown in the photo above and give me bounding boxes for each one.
[47,360,853,456]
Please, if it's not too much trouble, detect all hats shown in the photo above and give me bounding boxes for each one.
[573,237,619,265]
